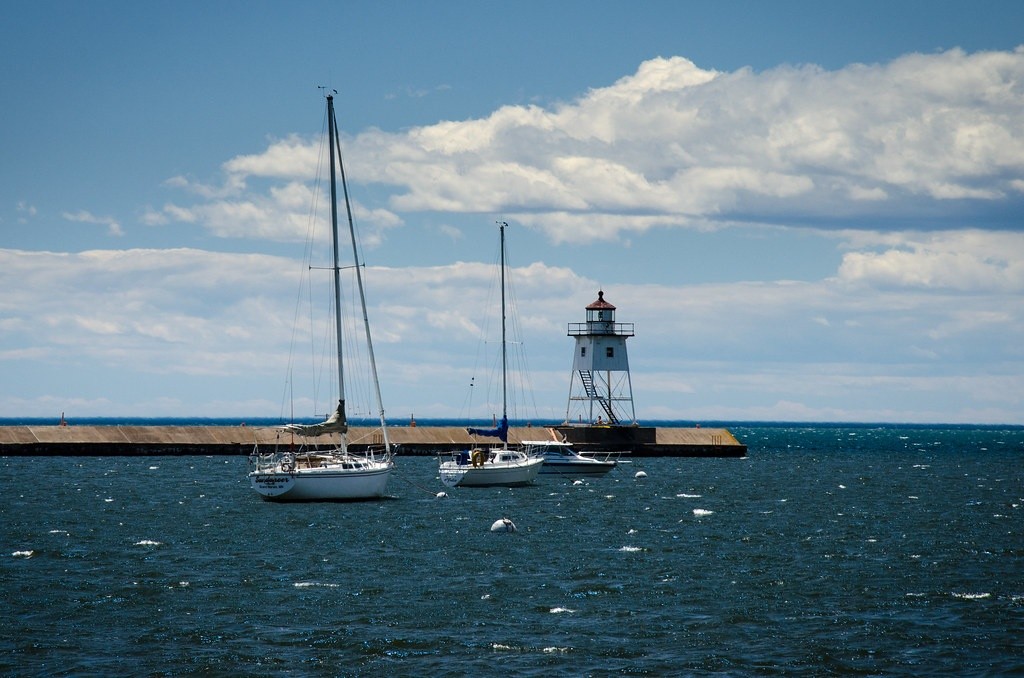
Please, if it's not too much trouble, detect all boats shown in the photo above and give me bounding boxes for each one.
[520,440,634,478]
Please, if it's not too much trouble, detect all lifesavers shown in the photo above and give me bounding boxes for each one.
[281,462,291,472]
[472,451,484,467]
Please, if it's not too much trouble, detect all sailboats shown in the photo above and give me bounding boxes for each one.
[245,85,400,502]
[436,219,545,486]
